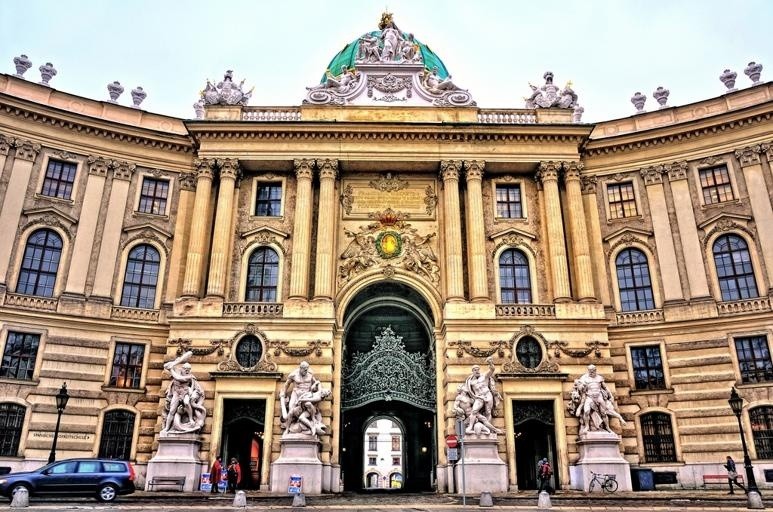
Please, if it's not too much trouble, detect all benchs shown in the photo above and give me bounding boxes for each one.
[147,476,186,492]
[703,475,745,488]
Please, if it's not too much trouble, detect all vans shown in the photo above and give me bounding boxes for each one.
[0,458,136,503]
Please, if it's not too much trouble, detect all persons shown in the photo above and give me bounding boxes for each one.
[226,457,240,494]
[574,364,614,435]
[373,22,406,62]
[216,70,237,100]
[297,381,332,438]
[540,457,555,495]
[422,66,455,94]
[357,33,380,61]
[398,33,419,64]
[464,357,495,433]
[453,385,505,435]
[279,362,316,436]
[160,361,194,431]
[304,65,355,91]
[396,234,434,283]
[723,455,747,495]
[208,455,223,493]
[540,71,559,105]
[339,232,379,281]
[535,460,550,494]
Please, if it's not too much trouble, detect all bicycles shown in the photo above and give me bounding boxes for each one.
[589,471,618,493]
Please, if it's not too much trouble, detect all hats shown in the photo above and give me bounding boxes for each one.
[231,458,237,463]
[543,458,547,461]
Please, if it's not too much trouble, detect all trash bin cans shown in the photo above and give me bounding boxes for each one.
[0,467,11,476]
[630,468,655,491]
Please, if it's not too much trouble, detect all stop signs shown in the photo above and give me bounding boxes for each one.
[446,436,457,447]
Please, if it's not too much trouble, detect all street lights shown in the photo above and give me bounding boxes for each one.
[728,386,762,496]
[46,382,70,465]
